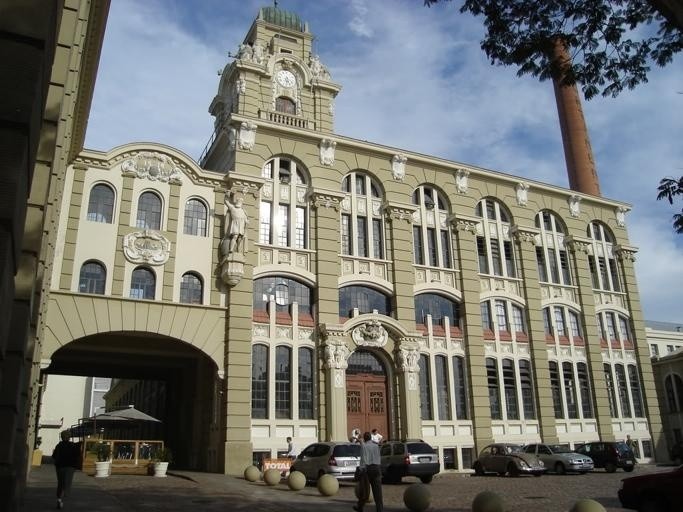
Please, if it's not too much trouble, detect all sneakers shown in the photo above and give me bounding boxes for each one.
[353,505,363,512]
[58,498,63,507]
[281,473,285,477]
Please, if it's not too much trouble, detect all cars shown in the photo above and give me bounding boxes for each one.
[380,439,439,484]
[521,443,594,475]
[473,443,547,478]
[574,442,634,473]
[289,441,360,484]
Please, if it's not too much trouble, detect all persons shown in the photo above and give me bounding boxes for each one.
[626,435,638,464]
[352,432,384,511]
[52,430,82,509]
[281,437,296,476]
[350,429,363,445]
[370,429,383,445]
[224,190,249,256]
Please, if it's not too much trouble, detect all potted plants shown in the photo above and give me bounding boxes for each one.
[31,437,43,467]
[88,442,114,477]
[150,447,174,478]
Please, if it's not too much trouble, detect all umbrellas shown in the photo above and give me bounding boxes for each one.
[75,400,165,440]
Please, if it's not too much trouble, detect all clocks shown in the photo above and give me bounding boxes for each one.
[275,69,297,89]
